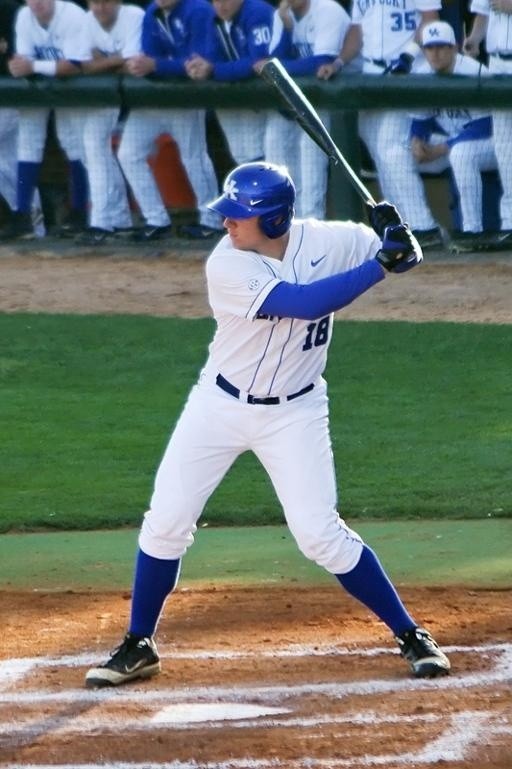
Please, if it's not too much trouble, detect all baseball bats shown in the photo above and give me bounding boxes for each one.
[259,57,417,263]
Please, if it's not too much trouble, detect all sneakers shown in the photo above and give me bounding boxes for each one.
[87,631,161,684]
[393,627,451,676]
[412,226,511,255]
[1,210,222,245]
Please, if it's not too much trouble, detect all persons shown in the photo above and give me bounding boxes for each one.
[85,162,452,688]
[1,0,512,254]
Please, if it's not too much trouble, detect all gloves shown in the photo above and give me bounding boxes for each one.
[365,202,401,237]
[375,224,415,271]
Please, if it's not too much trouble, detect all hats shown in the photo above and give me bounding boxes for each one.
[422,21,457,47]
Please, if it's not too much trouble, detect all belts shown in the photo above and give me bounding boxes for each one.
[491,51,512,61]
[217,374,314,405]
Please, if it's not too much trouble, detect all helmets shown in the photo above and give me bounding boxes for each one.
[208,163,295,239]
